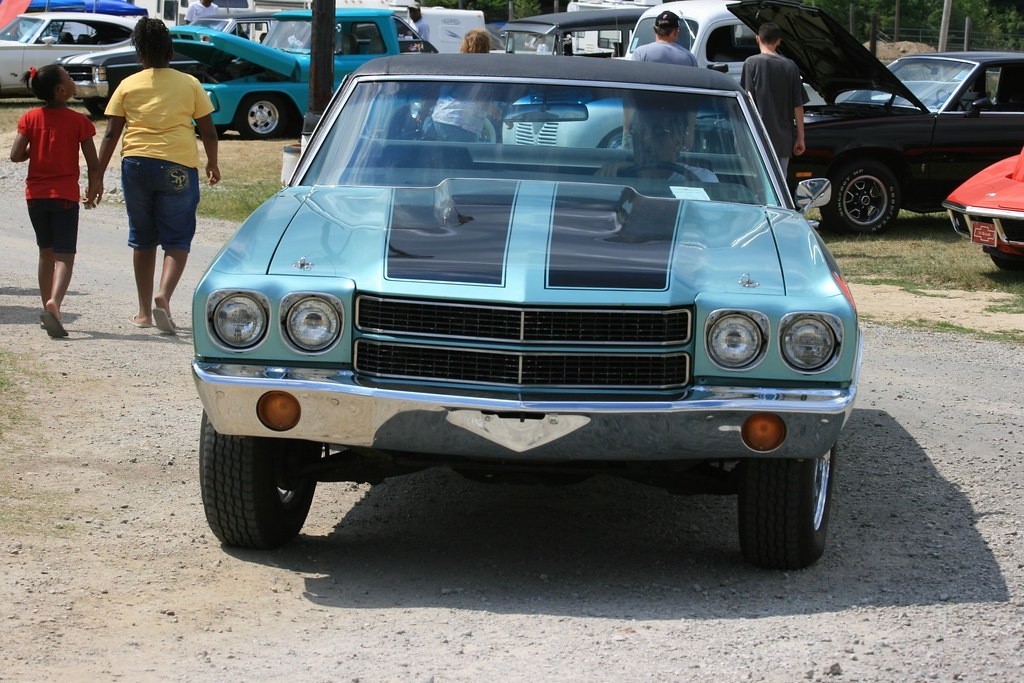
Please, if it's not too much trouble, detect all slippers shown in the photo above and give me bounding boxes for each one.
[152,308,177,335]
[126,313,153,328]
[39,311,68,337]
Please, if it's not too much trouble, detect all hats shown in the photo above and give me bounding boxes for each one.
[655,11,681,28]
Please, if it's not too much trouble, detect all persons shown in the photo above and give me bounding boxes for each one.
[524,33,549,53]
[408,0,430,42]
[591,101,721,184]
[431,30,502,144]
[183,0,220,25]
[10,63,101,339]
[740,21,806,181]
[85,17,222,334]
[621,10,702,151]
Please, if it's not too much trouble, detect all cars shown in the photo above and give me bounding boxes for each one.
[503,0,839,149]
[0,12,138,98]
[706,0,1024,235]
[941,150,1024,269]
[288,15,437,62]
[162,8,438,138]
[493,8,650,57]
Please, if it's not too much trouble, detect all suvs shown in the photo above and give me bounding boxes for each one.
[51,12,283,116]
[193,53,859,570]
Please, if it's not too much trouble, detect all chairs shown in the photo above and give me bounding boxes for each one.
[59,32,74,44]
[78,34,93,44]
[336,32,358,54]
[380,144,475,171]
[368,32,387,53]
[260,33,267,44]
[935,90,988,110]
